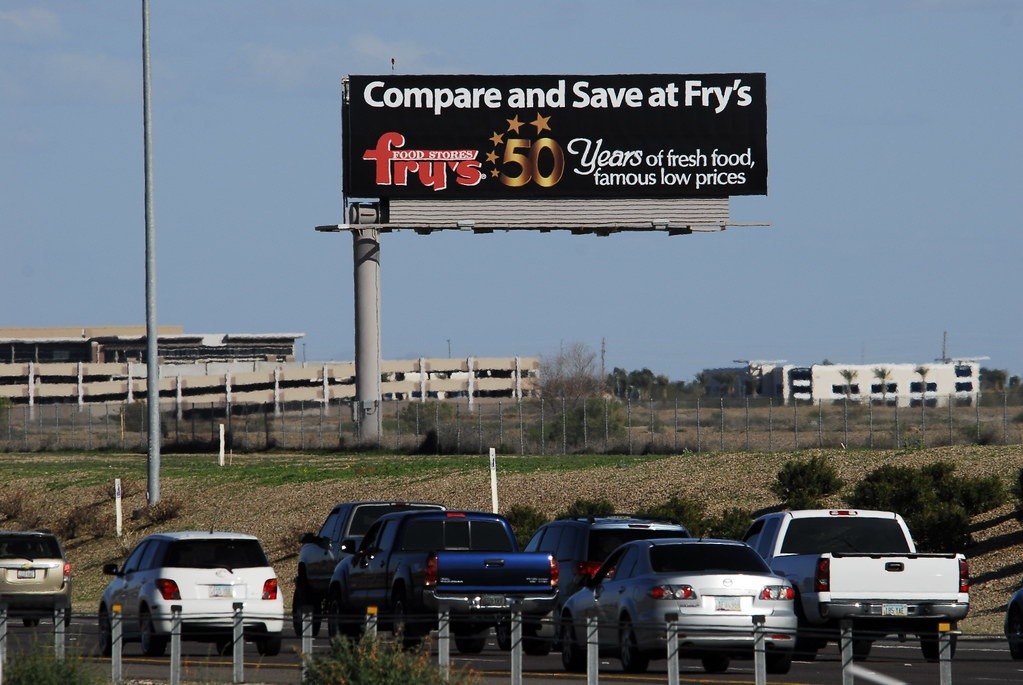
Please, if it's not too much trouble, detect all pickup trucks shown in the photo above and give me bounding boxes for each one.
[740,508,971,663]
[326,509,561,656]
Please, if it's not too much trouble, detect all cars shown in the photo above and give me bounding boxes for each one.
[0,530,73,626]
[560,538,798,675]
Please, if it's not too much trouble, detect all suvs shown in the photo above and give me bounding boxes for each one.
[521,514,694,655]
[99,530,285,657]
[293,502,447,639]
